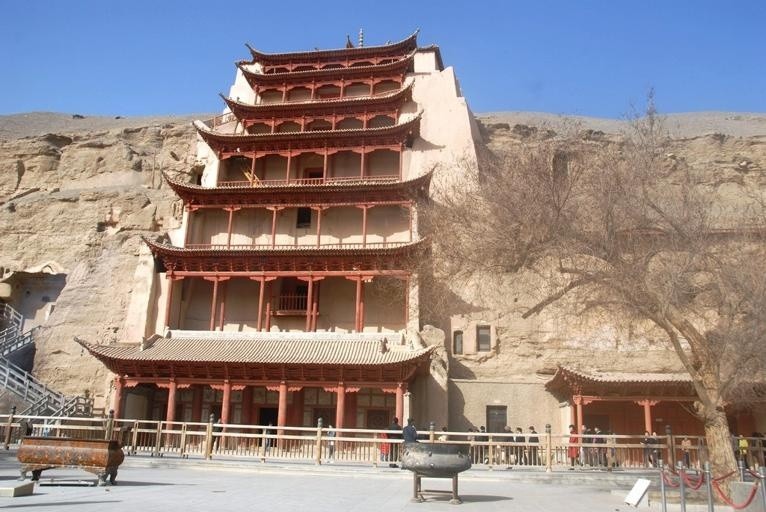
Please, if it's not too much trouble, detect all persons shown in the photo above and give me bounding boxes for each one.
[438,427,448,441]
[501,425,516,465]
[682,438,691,467]
[516,428,527,465]
[528,426,541,465]
[651,432,660,467]
[211,418,222,451]
[380,417,417,467]
[266,423,273,448]
[581,425,618,467]
[640,431,657,468]
[730,431,766,467]
[327,425,335,460]
[568,426,579,470]
[465,425,489,464]
